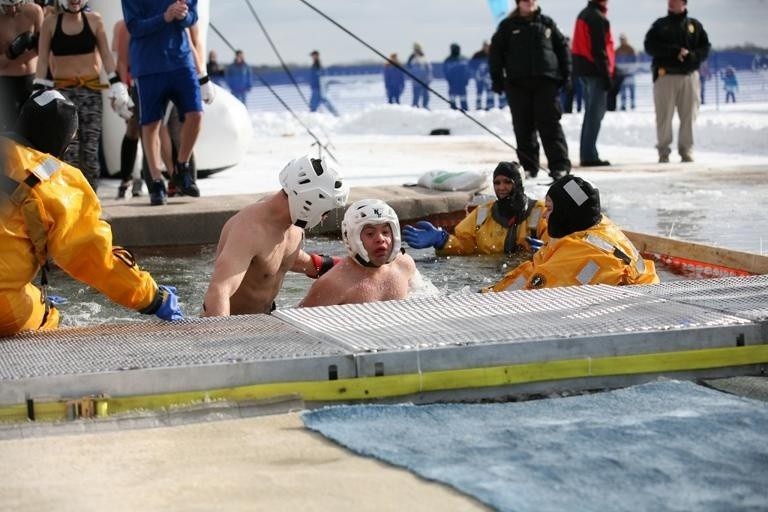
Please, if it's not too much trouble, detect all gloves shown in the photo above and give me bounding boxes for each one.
[5,30,35,62]
[400,220,448,250]
[155,284,185,319]
[524,234,544,252]
[45,293,70,306]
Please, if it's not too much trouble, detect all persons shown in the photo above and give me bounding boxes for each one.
[560,89,573,114]
[1,0,43,129]
[298,199,422,307]
[154,2,212,198]
[720,67,740,103]
[488,0,576,179]
[380,53,406,103]
[569,0,617,168]
[199,155,349,320]
[206,49,226,86]
[479,174,661,292]
[224,51,253,104]
[698,61,712,104]
[469,40,495,110]
[644,0,712,163]
[407,41,435,109]
[2,87,186,334]
[614,32,638,112]
[31,0,133,196]
[121,0,202,204]
[306,51,340,115]
[574,78,589,112]
[443,44,471,111]
[114,17,170,199]
[401,161,549,261]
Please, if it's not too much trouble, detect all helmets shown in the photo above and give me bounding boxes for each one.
[340,197,404,269]
[58,1,86,12]
[2,0,26,15]
[275,155,351,229]
[14,87,81,157]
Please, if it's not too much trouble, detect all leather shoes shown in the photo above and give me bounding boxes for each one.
[582,157,609,166]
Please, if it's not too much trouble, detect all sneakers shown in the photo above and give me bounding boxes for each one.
[149,178,165,205]
[681,155,693,161]
[172,159,203,197]
[659,155,668,162]
